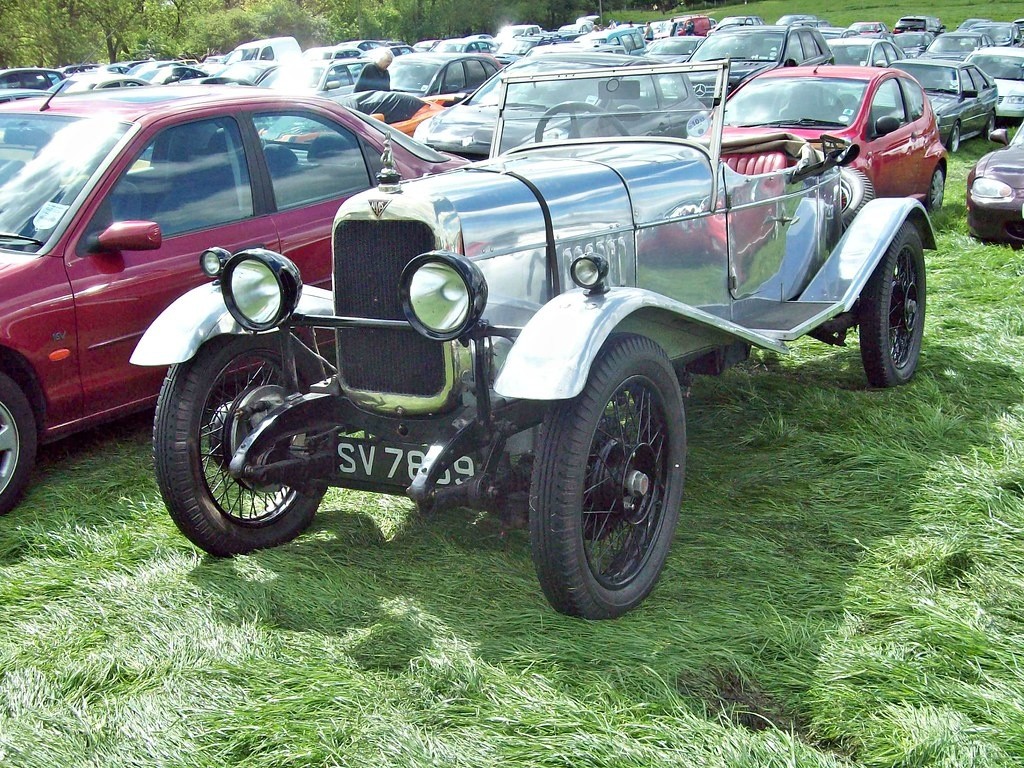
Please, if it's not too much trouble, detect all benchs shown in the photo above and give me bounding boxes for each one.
[719,151,787,177]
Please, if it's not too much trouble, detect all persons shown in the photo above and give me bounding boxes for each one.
[354,47,394,92]
[609,18,616,28]
[684,20,696,35]
[628,20,634,26]
[644,22,654,44]
[592,24,601,32]
[669,17,679,36]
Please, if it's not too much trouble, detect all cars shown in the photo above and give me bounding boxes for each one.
[412,53,708,163]
[0,84,473,517]
[683,65,949,241]
[126,60,937,621]
[967,119,1024,250]
[0,16,1024,153]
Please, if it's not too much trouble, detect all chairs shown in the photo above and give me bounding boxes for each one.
[59,138,141,222]
[778,92,816,119]
[207,128,368,204]
[150,153,240,236]
